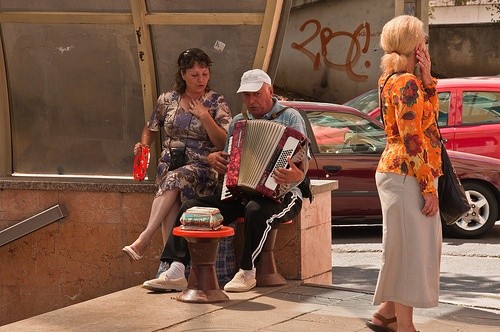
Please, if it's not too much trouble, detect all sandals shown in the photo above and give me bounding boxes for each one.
[366,312,397,332]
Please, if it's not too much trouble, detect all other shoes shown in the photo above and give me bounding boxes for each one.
[122,245,145,262]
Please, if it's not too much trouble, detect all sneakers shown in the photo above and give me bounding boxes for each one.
[141,273,188,292]
[223,272,257,292]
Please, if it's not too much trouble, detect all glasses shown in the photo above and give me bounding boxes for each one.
[183,49,204,58]
[424,35,430,45]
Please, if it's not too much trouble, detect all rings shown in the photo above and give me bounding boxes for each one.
[191,105,194,109]
[423,63,427,68]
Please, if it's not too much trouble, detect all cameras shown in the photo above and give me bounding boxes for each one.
[164,150,190,170]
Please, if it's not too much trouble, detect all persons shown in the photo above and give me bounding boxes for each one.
[121,48,233,279]
[141,69,310,291]
[366,16,442,332]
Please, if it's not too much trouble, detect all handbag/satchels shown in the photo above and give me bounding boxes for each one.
[437,145,472,226]
[162,150,189,171]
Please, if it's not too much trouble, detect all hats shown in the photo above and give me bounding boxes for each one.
[236,69,271,94]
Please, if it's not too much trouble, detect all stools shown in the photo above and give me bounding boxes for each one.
[236,216,292,287]
[173,225,235,303]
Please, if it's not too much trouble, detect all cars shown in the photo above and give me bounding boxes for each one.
[280,98,500,240]
[310,76,500,159]
